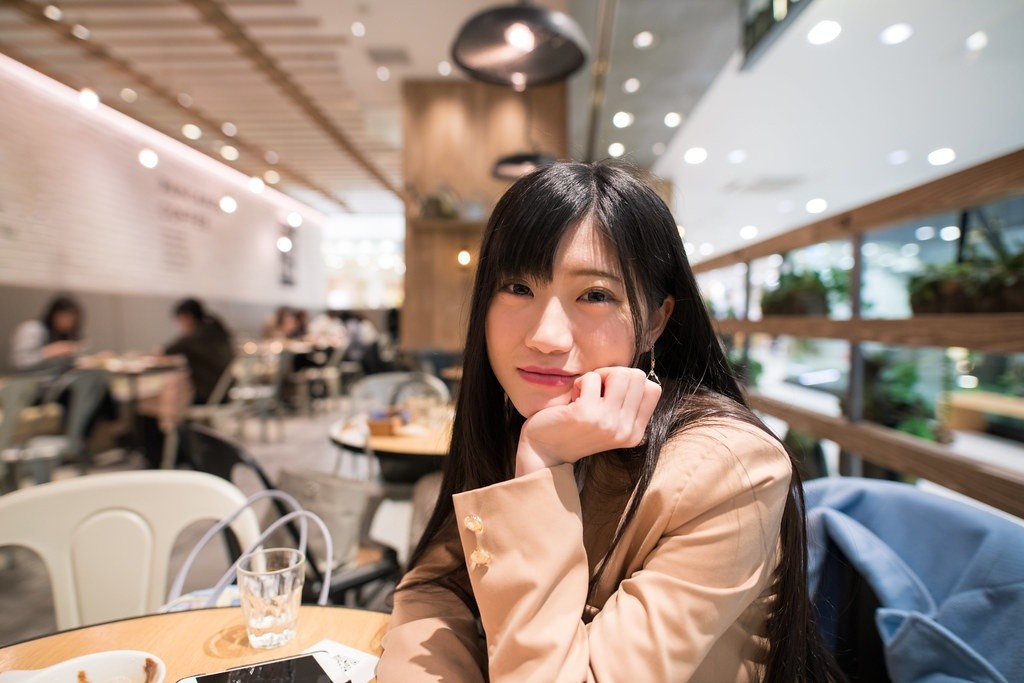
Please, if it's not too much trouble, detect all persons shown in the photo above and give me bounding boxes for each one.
[374,161,848,682]
[114,299,235,466]
[10,293,126,445]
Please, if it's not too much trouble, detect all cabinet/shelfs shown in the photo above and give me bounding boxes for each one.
[690,147,1023,521]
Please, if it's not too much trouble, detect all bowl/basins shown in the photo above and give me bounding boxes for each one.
[22,649,166,683]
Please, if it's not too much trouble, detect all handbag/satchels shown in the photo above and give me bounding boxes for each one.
[159,490,332,613]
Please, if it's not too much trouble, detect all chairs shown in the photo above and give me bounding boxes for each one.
[788,476,1024,683]
[0,335,461,651]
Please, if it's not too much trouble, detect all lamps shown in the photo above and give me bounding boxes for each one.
[451,0,592,95]
[493,83,564,183]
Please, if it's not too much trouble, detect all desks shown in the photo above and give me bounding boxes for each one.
[331,402,458,508]
[76,355,191,462]
[0,604,393,683]
[439,365,462,389]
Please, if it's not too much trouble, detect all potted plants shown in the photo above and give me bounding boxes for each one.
[703,219,1023,487]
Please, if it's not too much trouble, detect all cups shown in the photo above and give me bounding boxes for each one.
[405,397,435,425]
[236,546,306,651]
